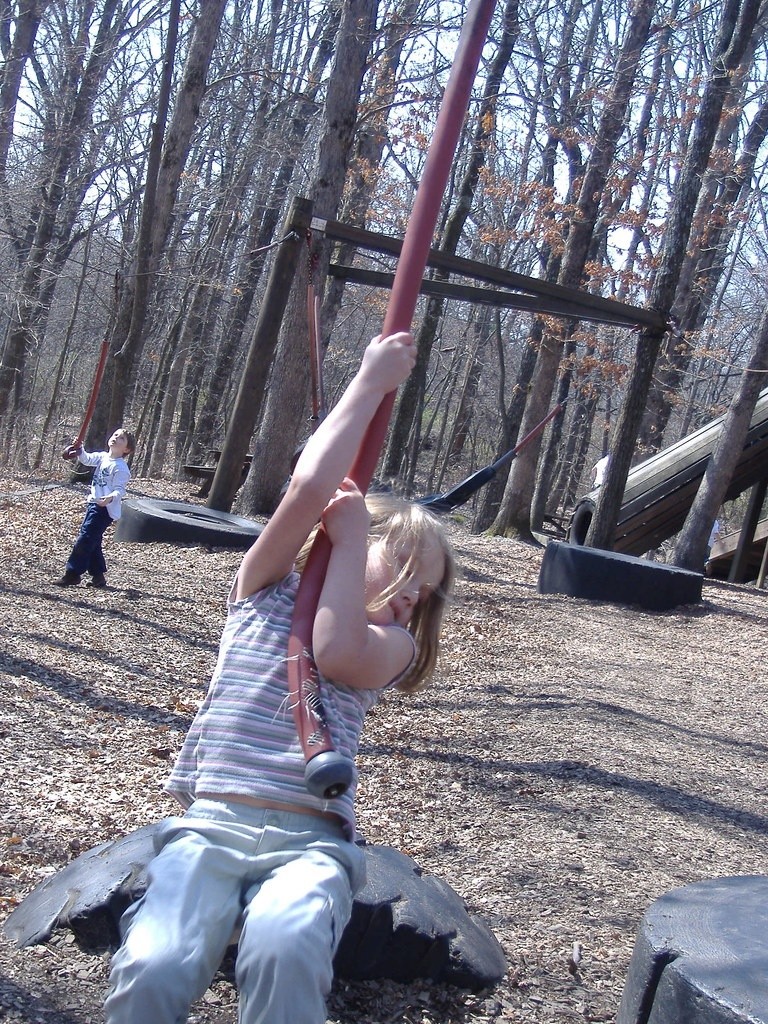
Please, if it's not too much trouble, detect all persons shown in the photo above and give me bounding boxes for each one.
[52,429,133,587]
[103,331,454,1024]
[705,518,726,561]
[590,451,609,488]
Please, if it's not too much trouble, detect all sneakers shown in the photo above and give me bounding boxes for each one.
[86,574,106,587]
[53,574,80,586]
